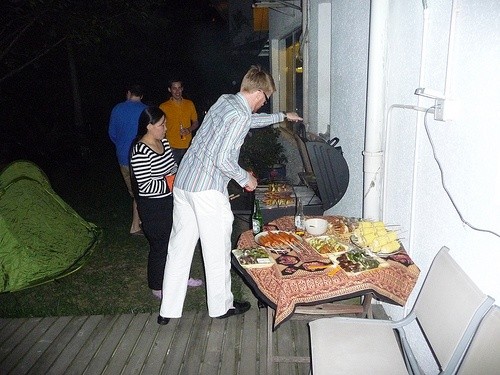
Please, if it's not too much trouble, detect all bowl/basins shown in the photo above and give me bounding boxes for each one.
[305,218,329,235]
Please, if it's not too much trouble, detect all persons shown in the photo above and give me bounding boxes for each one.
[158,80,199,165]
[158,65,304,324]
[129,108,202,298]
[109,82,151,234]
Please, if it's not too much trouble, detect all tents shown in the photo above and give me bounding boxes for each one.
[0,159,103,293]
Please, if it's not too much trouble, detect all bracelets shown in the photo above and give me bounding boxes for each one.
[283,111,287,120]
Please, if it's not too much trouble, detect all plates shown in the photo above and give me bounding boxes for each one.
[254,230,303,250]
[232,249,276,269]
[302,236,392,278]
[350,235,403,257]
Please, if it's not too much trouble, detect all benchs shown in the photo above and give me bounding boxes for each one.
[308,245,500,375]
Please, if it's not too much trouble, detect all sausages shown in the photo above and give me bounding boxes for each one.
[258,231,301,246]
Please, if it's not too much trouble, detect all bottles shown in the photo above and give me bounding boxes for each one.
[179,121,186,140]
[252,200,264,238]
[294,202,306,232]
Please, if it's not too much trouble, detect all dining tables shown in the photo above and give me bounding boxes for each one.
[239,214,377,321]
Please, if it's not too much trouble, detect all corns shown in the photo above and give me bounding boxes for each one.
[355,221,399,254]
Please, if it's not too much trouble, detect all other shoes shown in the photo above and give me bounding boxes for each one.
[152,289,162,301]
[188,275,203,286]
[157,314,171,325]
[128,230,145,237]
[216,300,251,319]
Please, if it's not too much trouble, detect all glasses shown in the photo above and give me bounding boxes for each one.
[258,90,270,105]
